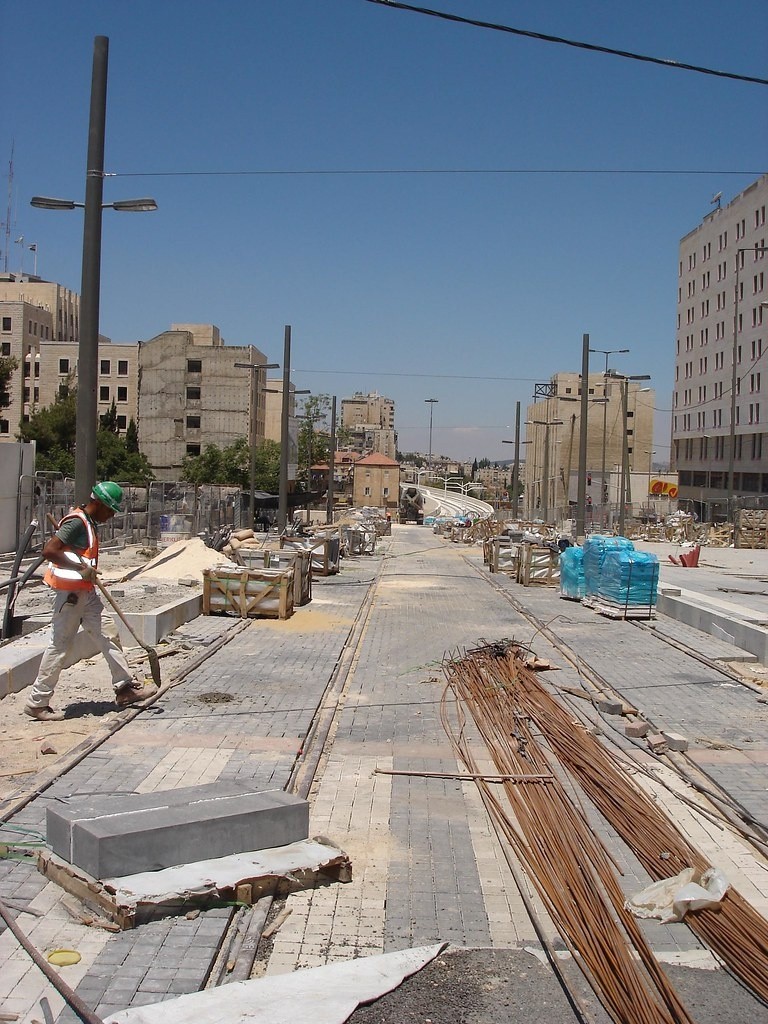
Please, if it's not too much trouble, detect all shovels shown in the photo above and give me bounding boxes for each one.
[46,512,163,689]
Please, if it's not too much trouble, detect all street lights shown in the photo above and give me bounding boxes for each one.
[604,373,651,536]
[589,349,630,533]
[29,193,157,510]
[233,363,281,532]
[727,247,768,525]
[404,469,488,512]
[533,421,564,522]
[260,388,311,534]
[559,396,610,545]
[294,414,327,527]
[595,380,651,507]
[501,440,533,518]
[424,398,438,470]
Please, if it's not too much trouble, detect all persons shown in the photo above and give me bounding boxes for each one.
[23,482,157,721]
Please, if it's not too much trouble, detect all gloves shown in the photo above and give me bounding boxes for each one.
[77,563,101,583]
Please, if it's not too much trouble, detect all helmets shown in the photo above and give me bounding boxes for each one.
[92,481,125,512]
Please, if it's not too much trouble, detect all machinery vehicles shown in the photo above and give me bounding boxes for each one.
[397,487,426,525]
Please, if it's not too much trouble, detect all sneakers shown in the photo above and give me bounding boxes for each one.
[115,682,157,706]
[24,705,64,721]
